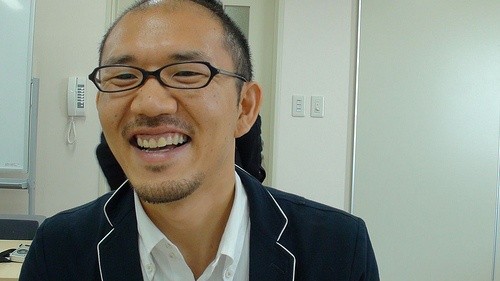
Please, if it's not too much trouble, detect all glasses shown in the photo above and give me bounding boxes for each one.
[88,60,248,93]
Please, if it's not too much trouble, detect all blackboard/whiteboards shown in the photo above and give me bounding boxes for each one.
[0,0,35,172]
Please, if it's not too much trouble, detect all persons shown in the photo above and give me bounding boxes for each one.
[18,0,381,281]
[95,114,267,190]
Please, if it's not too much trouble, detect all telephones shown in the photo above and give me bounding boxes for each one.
[67,75,88,117]
